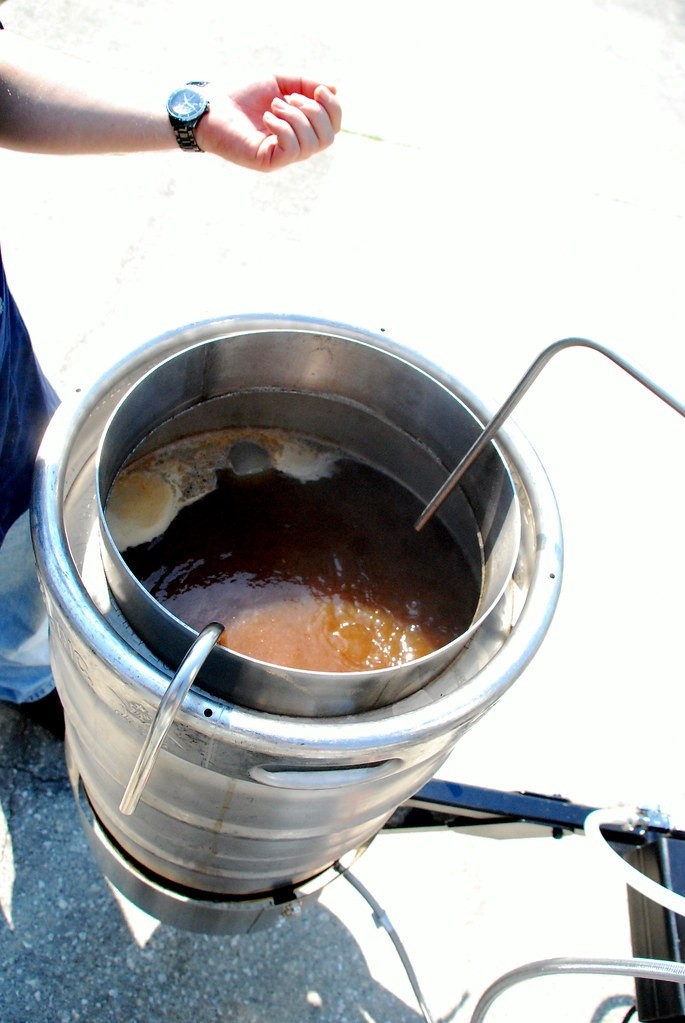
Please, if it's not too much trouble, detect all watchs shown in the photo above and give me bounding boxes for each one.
[165,79,210,152]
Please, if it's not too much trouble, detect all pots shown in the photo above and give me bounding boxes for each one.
[29,312,565,937]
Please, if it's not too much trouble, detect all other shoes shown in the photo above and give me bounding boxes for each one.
[0,687,65,742]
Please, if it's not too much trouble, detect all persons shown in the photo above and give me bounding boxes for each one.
[0,60,343,737]
